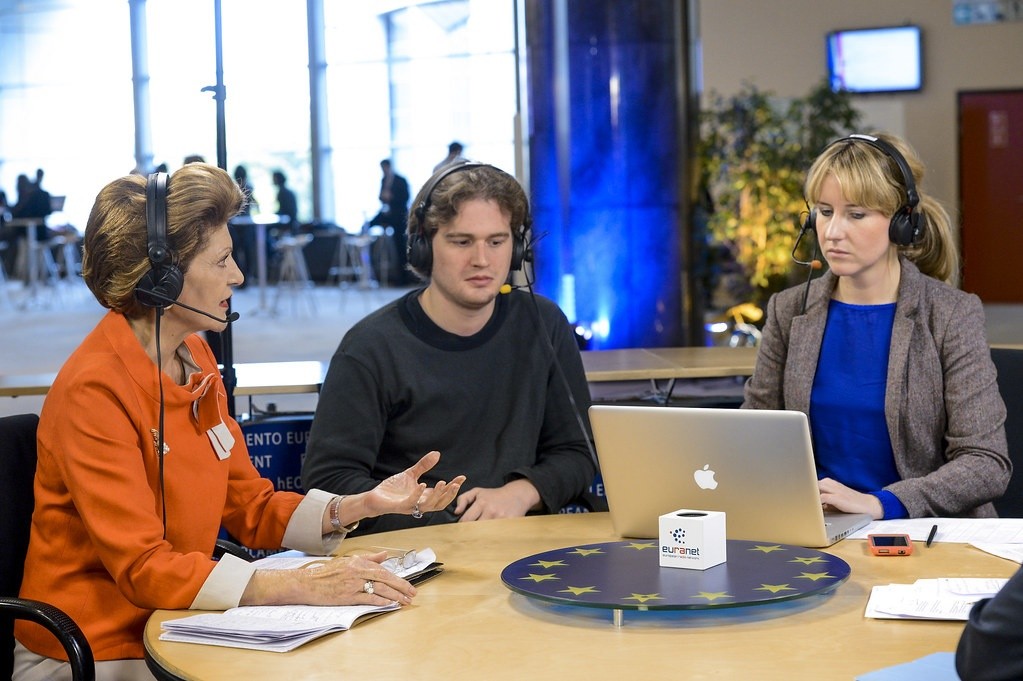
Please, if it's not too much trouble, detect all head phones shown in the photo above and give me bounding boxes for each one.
[806,134,928,246]
[407,162,528,272]
[133,171,183,308]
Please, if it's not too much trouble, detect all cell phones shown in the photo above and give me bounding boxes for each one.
[868,533,915,557]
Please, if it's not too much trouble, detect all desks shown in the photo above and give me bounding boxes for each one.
[0,361,324,419]
[143,511,1023,681]
[580,349,677,405]
[646,348,758,407]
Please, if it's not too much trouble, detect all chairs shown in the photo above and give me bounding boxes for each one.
[0,413,93,681]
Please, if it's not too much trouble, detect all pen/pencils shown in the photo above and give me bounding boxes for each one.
[927,525,937,547]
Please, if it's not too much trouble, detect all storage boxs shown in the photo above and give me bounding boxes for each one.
[658,508,727,571]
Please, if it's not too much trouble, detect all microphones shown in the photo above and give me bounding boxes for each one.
[134,288,240,323]
[791,216,821,268]
[501,251,535,294]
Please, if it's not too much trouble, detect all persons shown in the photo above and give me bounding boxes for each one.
[0,168,58,280]
[301,163,609,537]
[12,162,466,681]
[370,160,409,292]
[226,166,260,224]
[433,142,468,179]
[274,172,296,233]
[955,562,1023,681]
[184,157,205,166]
[738,133,1014,521]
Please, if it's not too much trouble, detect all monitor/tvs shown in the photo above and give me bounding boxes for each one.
[826,26,923,94]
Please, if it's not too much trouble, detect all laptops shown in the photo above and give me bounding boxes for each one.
[589,405,873,548]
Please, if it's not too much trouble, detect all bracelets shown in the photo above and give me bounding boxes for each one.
[330,495,359,533]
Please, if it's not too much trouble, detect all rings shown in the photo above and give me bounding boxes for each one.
[412,501,424,519]
[363,580,375,594]
